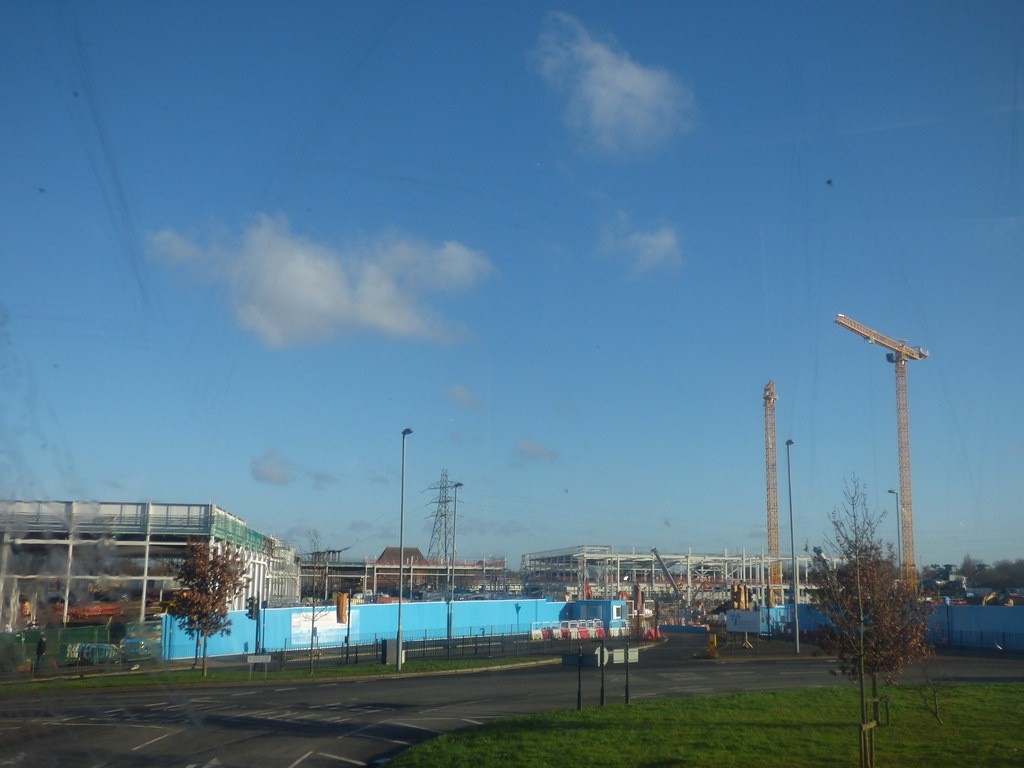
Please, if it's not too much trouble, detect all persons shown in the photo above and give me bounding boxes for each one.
[36,634,47,671]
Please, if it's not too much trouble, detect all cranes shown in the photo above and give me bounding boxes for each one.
[838,314,930,653]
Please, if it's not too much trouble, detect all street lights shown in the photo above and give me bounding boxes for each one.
[889,489,907,660]
[395,428,414,670]
[786,439,801,656]
[448,483,461,657]
